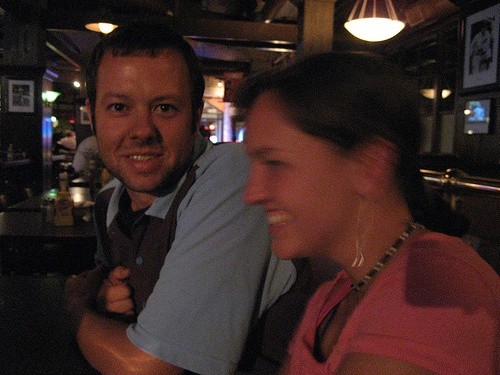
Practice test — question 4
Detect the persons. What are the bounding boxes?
[68,21,344,375]
[223,50,500,375]
[52,125,78,187]
[469,102,486,123]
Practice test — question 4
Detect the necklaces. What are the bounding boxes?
[298,221,424,336]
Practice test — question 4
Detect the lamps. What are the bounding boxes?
[344,0,405,43]
[84,14,120,35]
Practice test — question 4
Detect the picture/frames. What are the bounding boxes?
[2,75,40,119]
[455,3,500,94]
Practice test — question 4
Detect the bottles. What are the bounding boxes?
[7,143,14,161]
[53,170,74,227]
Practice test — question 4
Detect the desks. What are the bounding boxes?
[0,186,99,237]
[0,273,101,375]
